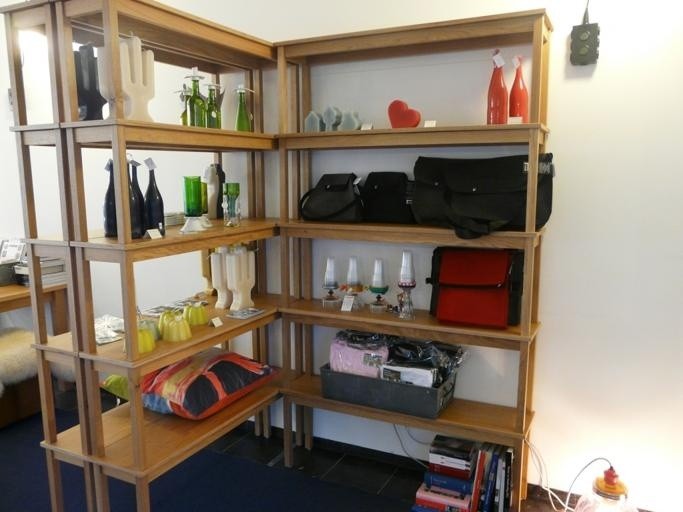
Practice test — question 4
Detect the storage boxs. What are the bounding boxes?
[320,362,457,421]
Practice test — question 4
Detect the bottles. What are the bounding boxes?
[488,51,529,125]
[179,67,254,132]
[104,159,165,242]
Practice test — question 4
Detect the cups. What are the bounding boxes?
[223,182,242,227]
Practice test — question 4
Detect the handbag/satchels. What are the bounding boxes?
[424,246,524,330]
[295,171,407,221]
[410,154,556,242]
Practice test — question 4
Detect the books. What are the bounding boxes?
[410,432,514,512]
[13,256,67,288]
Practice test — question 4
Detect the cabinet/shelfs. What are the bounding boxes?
[270,5,554,512]
[0,4,290,511]
